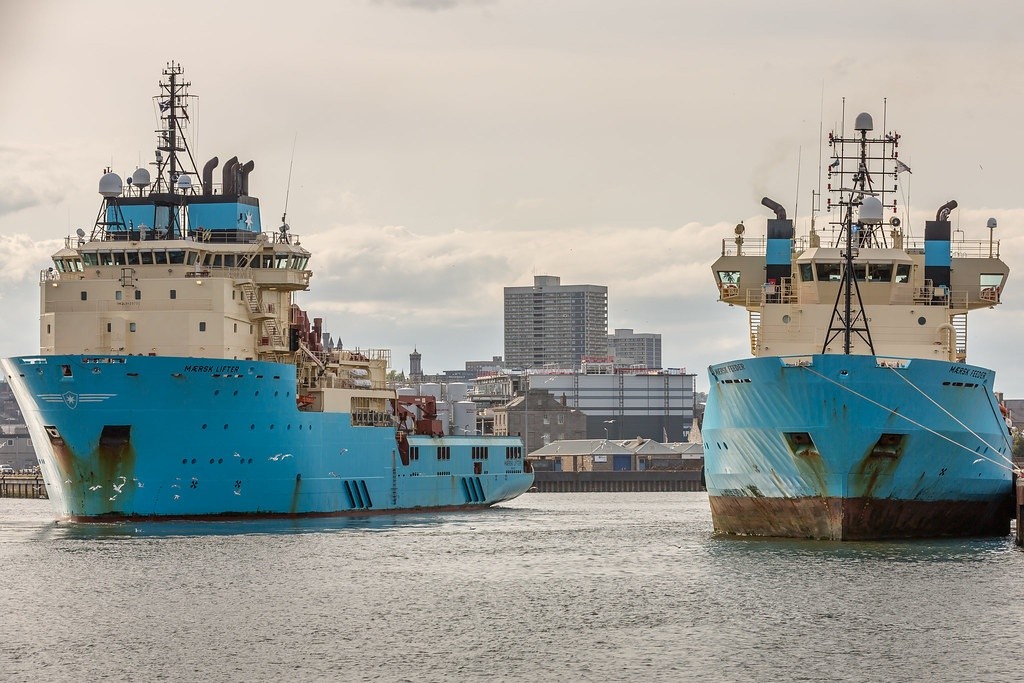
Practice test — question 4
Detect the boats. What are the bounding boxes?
[702,95,1017,544]
[0,55,536,524]
